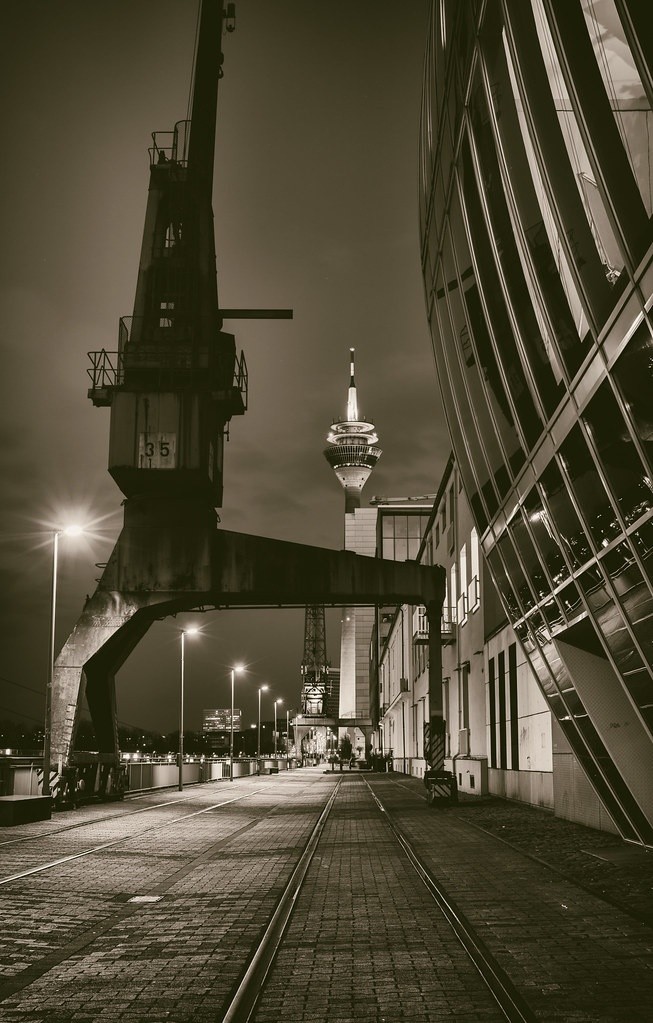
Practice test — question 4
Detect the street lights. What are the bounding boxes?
[296,714,302,751]
[286,709,293,771]
[42,528,85,795]
[274,700,282,766]
[230,666,243,781]
[258,686,268,775]
[179,628,197,791]
